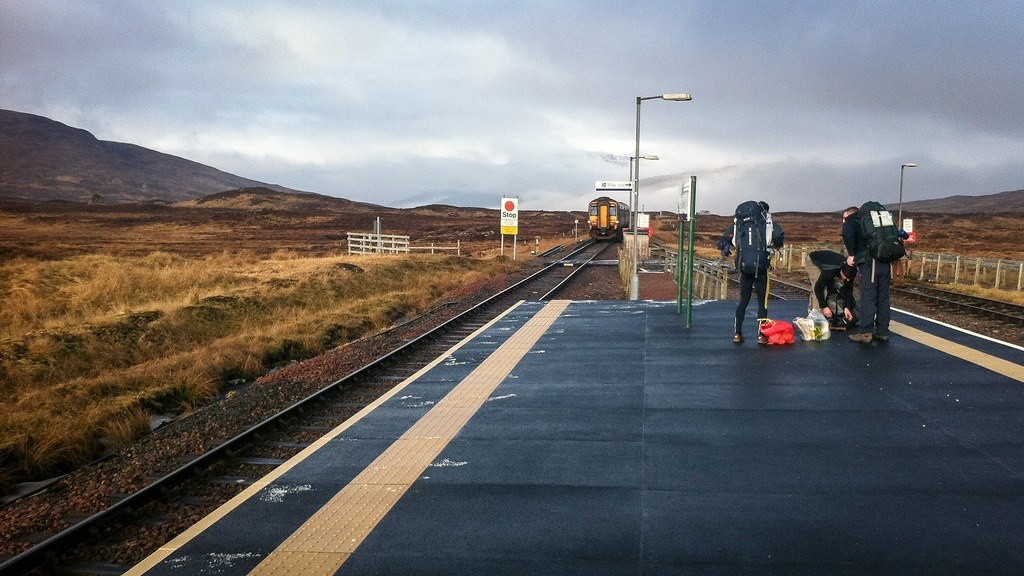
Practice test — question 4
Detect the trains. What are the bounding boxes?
[587,197,631,241]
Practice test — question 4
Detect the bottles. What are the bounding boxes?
[898,227,909,240]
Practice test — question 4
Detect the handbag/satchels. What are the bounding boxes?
[759,318,794,345]
[792,309,831,341]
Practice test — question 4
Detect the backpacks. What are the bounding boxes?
[844,201,907,262]
[732,200,773,278]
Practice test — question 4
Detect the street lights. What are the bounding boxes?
[628,155,661,233]
[628,93,693,301]
[897,163,917,231]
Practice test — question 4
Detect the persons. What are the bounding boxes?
[716,201,783,346]
[843,206,892,342]
[808,249,858,327]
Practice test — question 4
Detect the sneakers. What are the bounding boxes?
[848,332,872,344]
[732,334,745,342]
[872,333,889,341]
[757,335,768,344]
